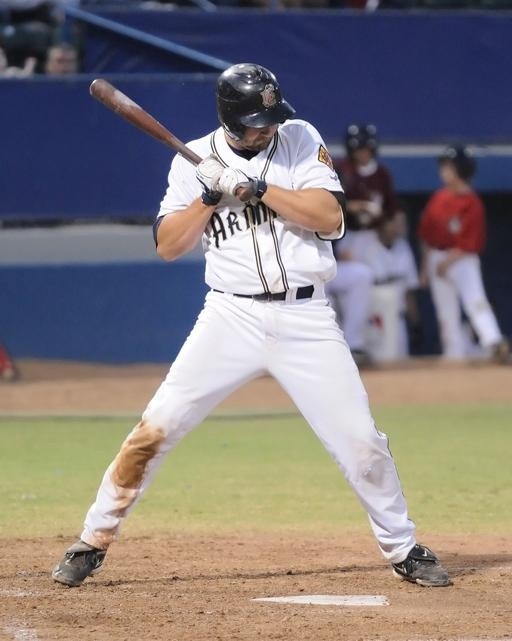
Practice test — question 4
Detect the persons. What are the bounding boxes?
[52,63,452,588]
[0,1,84,77]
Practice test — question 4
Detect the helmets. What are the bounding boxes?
[344,123,476,182]
[215,62,296,143]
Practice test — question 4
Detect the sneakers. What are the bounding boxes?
[51,538,108,588]
[391,543,451,588]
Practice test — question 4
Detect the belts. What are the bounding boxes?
[212,283,316,303]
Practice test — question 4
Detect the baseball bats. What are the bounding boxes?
[89,78,252,201]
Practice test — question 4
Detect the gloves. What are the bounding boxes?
[215,165,258,197]
[195,152,225,195]
